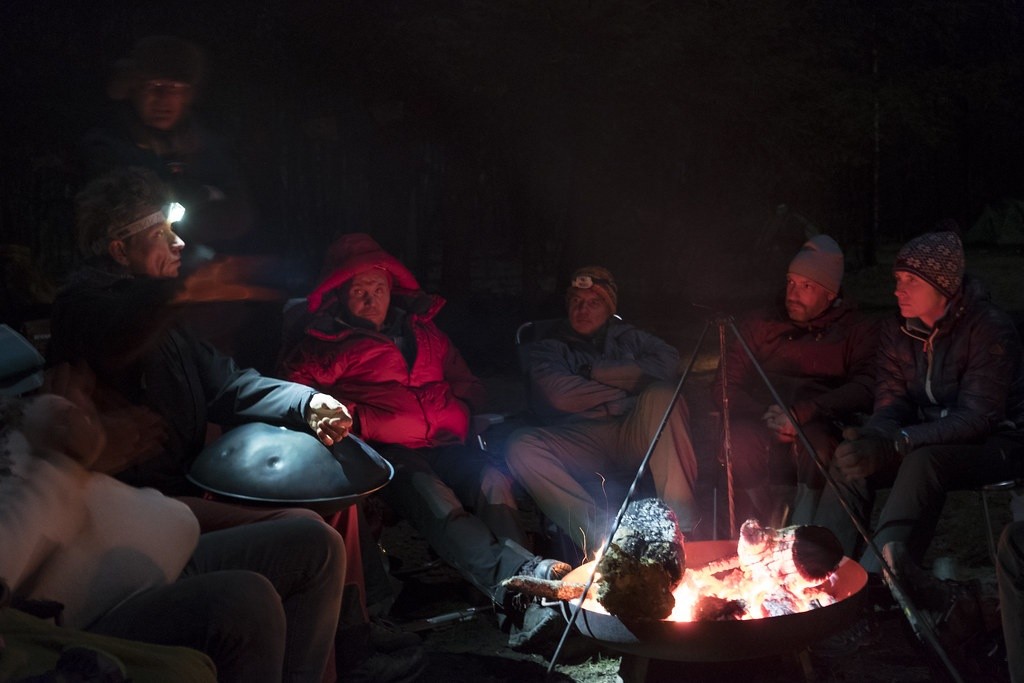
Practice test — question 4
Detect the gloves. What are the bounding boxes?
[836,426,902,483]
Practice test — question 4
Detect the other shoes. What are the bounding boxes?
[806,602,893,658]
[508,591,591,663]
[333,616,425,683]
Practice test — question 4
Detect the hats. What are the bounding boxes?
[109,35,204,102]
[892,232,964,299]
[787,235,845,297]
[567,264,617,317]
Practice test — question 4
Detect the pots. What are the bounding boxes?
[560,540,868,658]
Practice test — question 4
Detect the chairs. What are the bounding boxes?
[284,296,446,577]
[513,315,657,567]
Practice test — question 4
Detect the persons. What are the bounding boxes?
[995,520,1024,683]
[811,230,1024,658]
[712,234,881,531]
[0,37,428,683]
[281,233,572,646]
[504,264,701,566]
